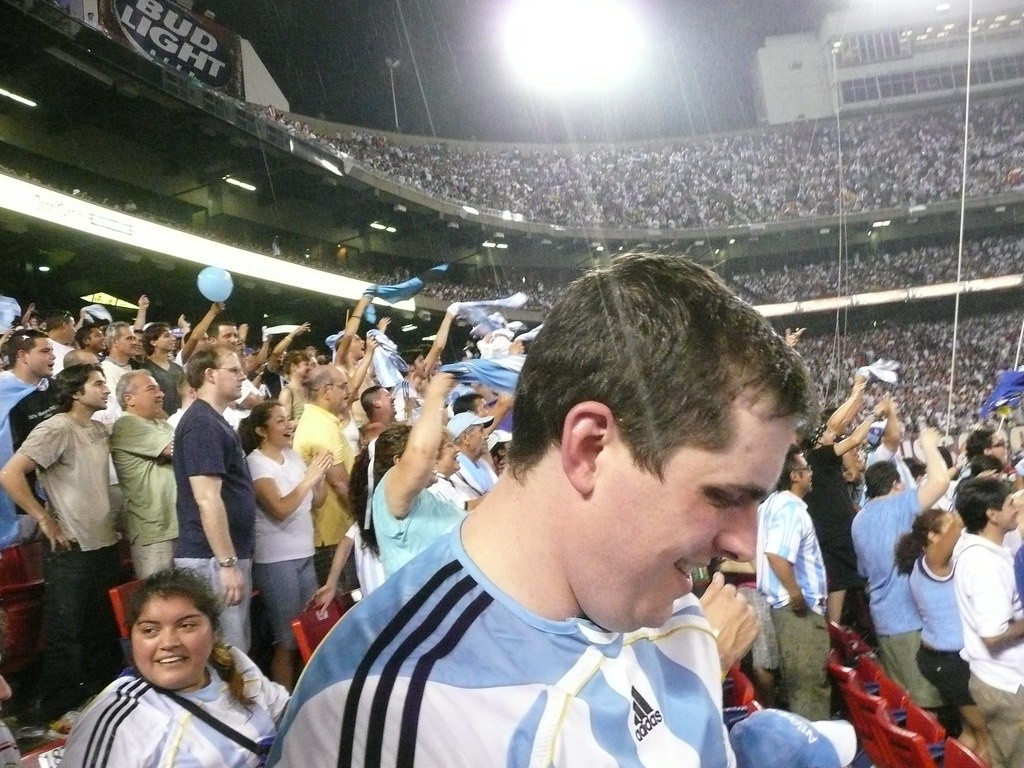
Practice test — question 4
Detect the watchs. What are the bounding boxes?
[220,556,239,567]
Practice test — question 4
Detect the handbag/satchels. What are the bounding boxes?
[256,736,275,768]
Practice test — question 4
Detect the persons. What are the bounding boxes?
[265,240,823,768]
[0,0,1023,768]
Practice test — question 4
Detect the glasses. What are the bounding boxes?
[326,383,348,390]
[22,330,38,341]
[790,465,811,473]
[64,310,72,318]
[212,367,243,377]
[991,439,1005,447]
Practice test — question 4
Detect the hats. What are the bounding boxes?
[487,430,513,451]
[447,413,494,441]
[729,708,857,768]
[867,418,887,446]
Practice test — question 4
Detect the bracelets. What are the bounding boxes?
[863,409,878,424]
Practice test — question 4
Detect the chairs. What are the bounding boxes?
[0,542,146,669]
[727,621,991,768]
[290,595,341,665]
